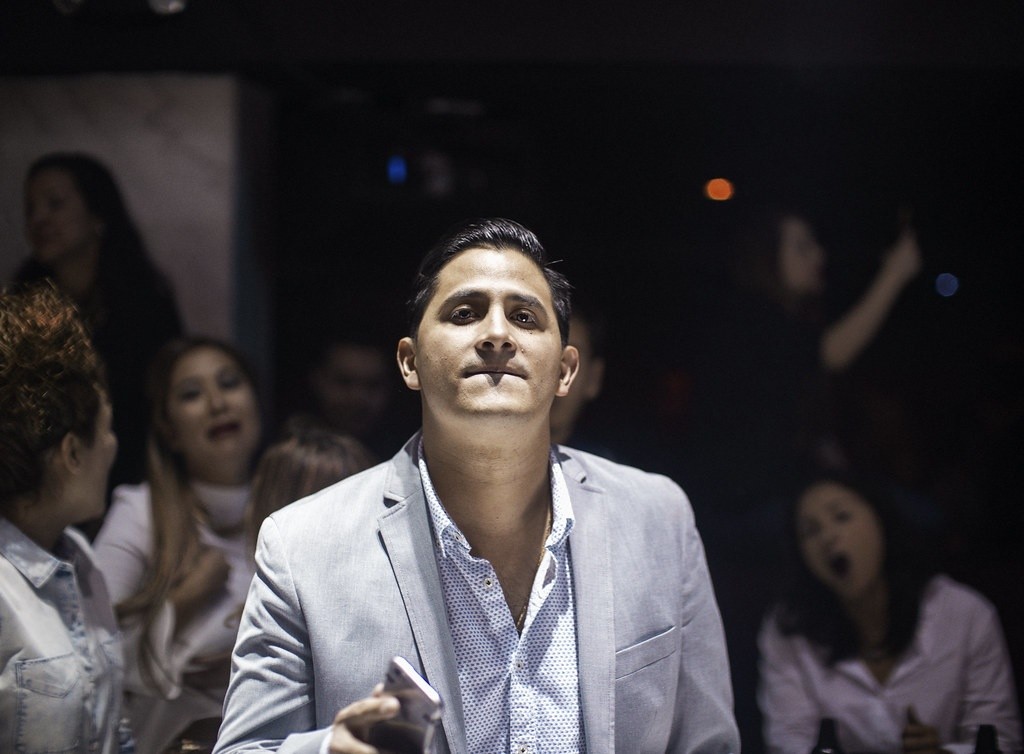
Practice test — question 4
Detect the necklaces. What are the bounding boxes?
[514,506,552,625]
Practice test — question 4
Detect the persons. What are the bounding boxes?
[214,220,742,754]
[16,151,179,505]
[0,281,601,754]
[729,205,1024,753]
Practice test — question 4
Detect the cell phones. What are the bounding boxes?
[366,657,441,754]
